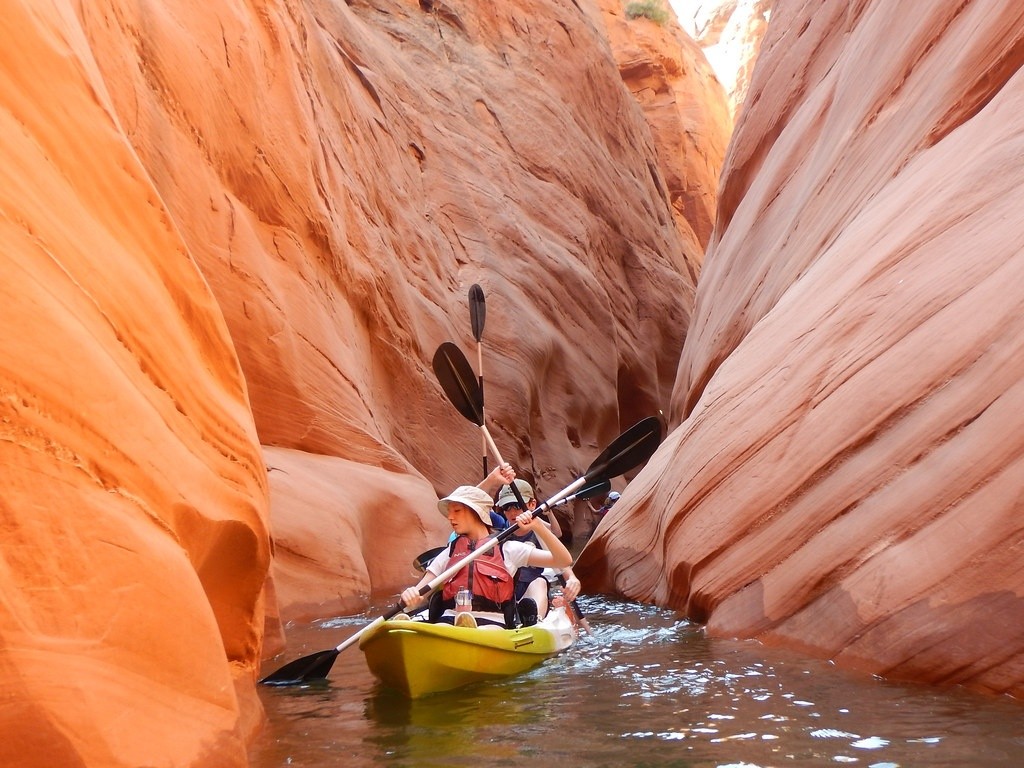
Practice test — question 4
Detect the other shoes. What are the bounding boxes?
[455,611,477,628]
[518,597,538,626]
[392,613,410,620]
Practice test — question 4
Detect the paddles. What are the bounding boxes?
[410,478,612,574]
[468,282,490,479]
[431,340,595,639]
[258,415,663,689]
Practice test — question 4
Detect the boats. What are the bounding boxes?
[357,593,579,700]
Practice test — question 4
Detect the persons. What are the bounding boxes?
[586,491,620,515]
[398,478,582,629]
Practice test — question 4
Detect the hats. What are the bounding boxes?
[499,478,534,507]
[609,491,620,501]
[495,490,503,506]
[437,486,494,526]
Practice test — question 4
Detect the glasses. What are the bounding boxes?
[502,502,530,511]
